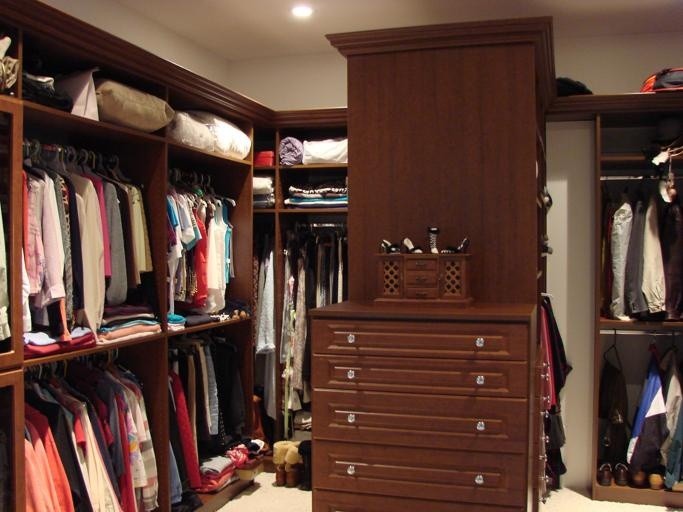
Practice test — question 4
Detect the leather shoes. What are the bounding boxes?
[599,463,664,489]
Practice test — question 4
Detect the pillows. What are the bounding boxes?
[94,78,176,133]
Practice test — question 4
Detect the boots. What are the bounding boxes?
[273,440,311,490]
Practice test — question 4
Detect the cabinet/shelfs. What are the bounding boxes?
[308,320,530,511]
[253,124,348,472]
[320,14,551,512]
[1,25,255,512]
[593,115,683,508]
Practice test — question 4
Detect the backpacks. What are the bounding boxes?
[641,68,683,91]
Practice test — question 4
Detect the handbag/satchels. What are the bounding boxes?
[61,68,101,121]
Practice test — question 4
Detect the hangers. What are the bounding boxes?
[169,165,215,193]
[22,137,121,177]
[24,347,119,385]
[610,328,675,356]
[174,331,226,349]
[603,152,677,199]
[286,222,347,248]
